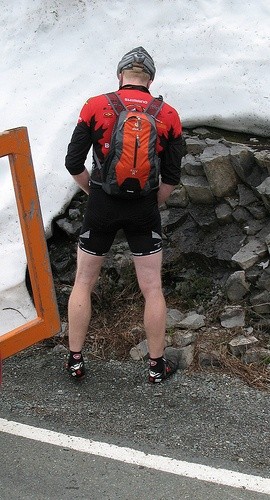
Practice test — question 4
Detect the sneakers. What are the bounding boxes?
[67,353,87,380]
[146,352,176,383]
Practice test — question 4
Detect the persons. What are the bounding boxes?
[65,46,188,383]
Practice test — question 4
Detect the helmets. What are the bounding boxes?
[116,46,156,82]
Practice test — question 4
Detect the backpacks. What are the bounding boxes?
[94,93,165,197]
